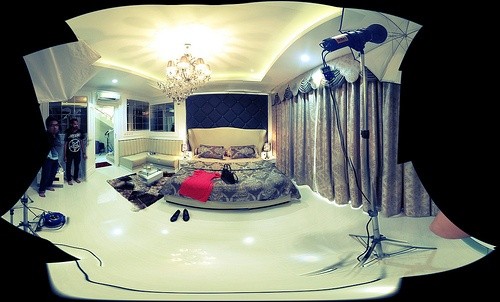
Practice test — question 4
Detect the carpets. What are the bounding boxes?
[96,162,112,169]
[106,173,175,210]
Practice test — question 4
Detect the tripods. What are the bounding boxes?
[347,43,439,278]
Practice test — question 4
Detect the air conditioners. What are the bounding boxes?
[97,90,120,102]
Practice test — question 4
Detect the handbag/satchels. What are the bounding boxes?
[221,163,238,184]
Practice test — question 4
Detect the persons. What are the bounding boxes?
[39,115,62,197]
[63,118,88,186]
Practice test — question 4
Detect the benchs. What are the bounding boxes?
[118,138,183,172]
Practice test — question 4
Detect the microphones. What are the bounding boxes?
[323,24,387,53]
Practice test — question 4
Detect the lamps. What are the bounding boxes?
[157,42,212,105]
[181,144,187,158]
[264,143,271,159]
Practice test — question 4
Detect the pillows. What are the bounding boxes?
[229,144,257,159]
[198,144,224,160]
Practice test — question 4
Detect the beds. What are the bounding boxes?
[163,127,302,210]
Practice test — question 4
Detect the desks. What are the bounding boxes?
[137,169,163,185]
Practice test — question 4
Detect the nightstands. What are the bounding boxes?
[261,155,276,165]
[178,159,189,170]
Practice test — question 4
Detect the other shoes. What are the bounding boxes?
[170,210,180,222]
[183,209,189,221]
